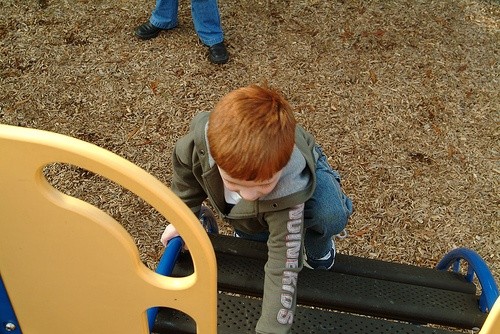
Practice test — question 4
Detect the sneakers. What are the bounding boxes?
[303,239,335,270]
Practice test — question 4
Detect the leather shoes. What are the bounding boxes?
[199,38,229,64]
[135,20,162,40]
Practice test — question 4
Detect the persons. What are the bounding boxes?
[161,84,353,334]
[135,0,229,64]
